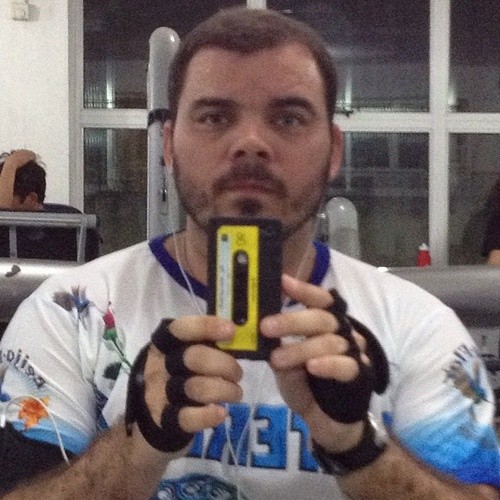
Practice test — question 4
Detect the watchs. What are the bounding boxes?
[309,411,388,477]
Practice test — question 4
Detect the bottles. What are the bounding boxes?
[417,242,430,266]
[478,336,500,424]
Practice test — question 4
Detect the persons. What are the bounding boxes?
[487,180,500,271]
[0,148,102,264]
[0,6,500,500]
